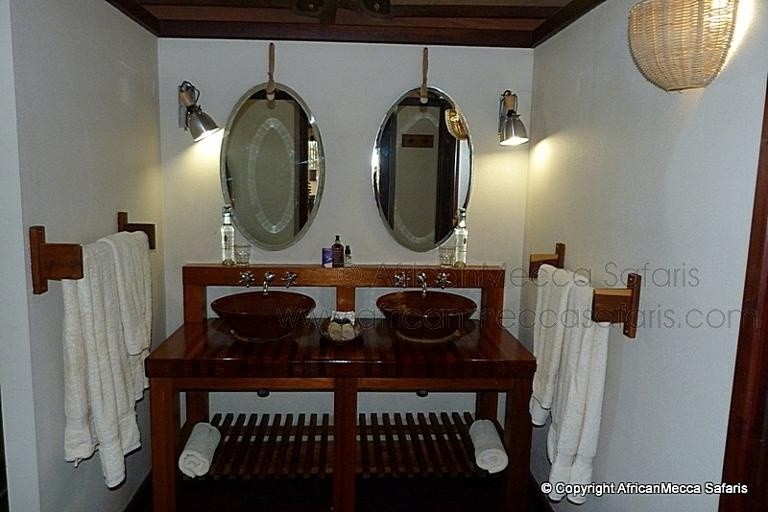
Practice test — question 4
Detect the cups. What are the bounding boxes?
[438,246,455,268]
[234,244,251,264]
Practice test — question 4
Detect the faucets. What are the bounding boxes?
[262,269,275,297]
[416,271,428,298]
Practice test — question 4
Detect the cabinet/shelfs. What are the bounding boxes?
[144,317,537,512]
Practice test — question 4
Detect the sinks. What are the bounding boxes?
[210,292,315,341]
[376,291,477,342]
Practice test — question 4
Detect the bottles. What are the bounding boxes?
[330,235,352,268]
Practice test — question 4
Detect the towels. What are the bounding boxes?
[178,422,221,478]
[60,242,143,489]
[469,420,509,474]
[97,231,153,401]
[529,264,590,426]
[547,283,611,504]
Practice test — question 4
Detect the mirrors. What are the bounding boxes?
[372,86,472,252]
[219,83,326,250]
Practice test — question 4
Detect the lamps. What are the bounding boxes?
[178,80,219,142]
[444,109,467,140]
[499,90,528,147]
